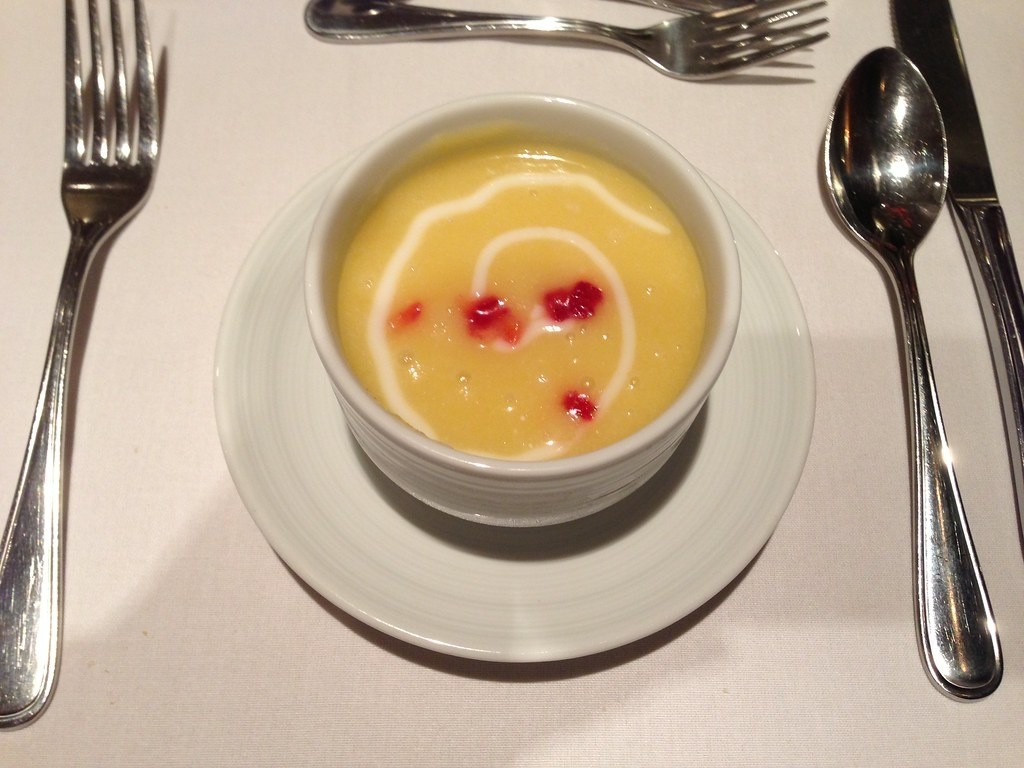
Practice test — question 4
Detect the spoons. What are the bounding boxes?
[821,46,1005,705]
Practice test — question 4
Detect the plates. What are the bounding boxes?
[211,141,817,664]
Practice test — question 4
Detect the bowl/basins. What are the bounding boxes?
[303,92,743,527]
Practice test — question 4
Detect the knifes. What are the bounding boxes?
[889,0,1024,564]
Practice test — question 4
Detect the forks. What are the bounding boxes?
[303,1,831,82]
[0,1,163,731]
